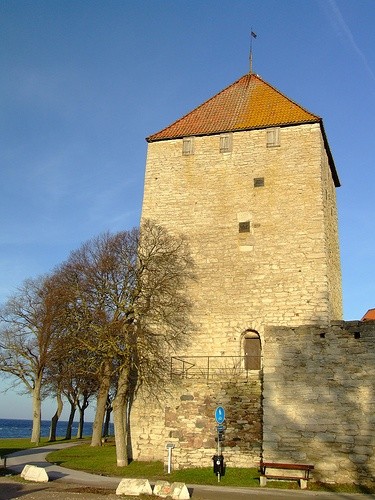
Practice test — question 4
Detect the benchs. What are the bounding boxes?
[256,461,314,490]
